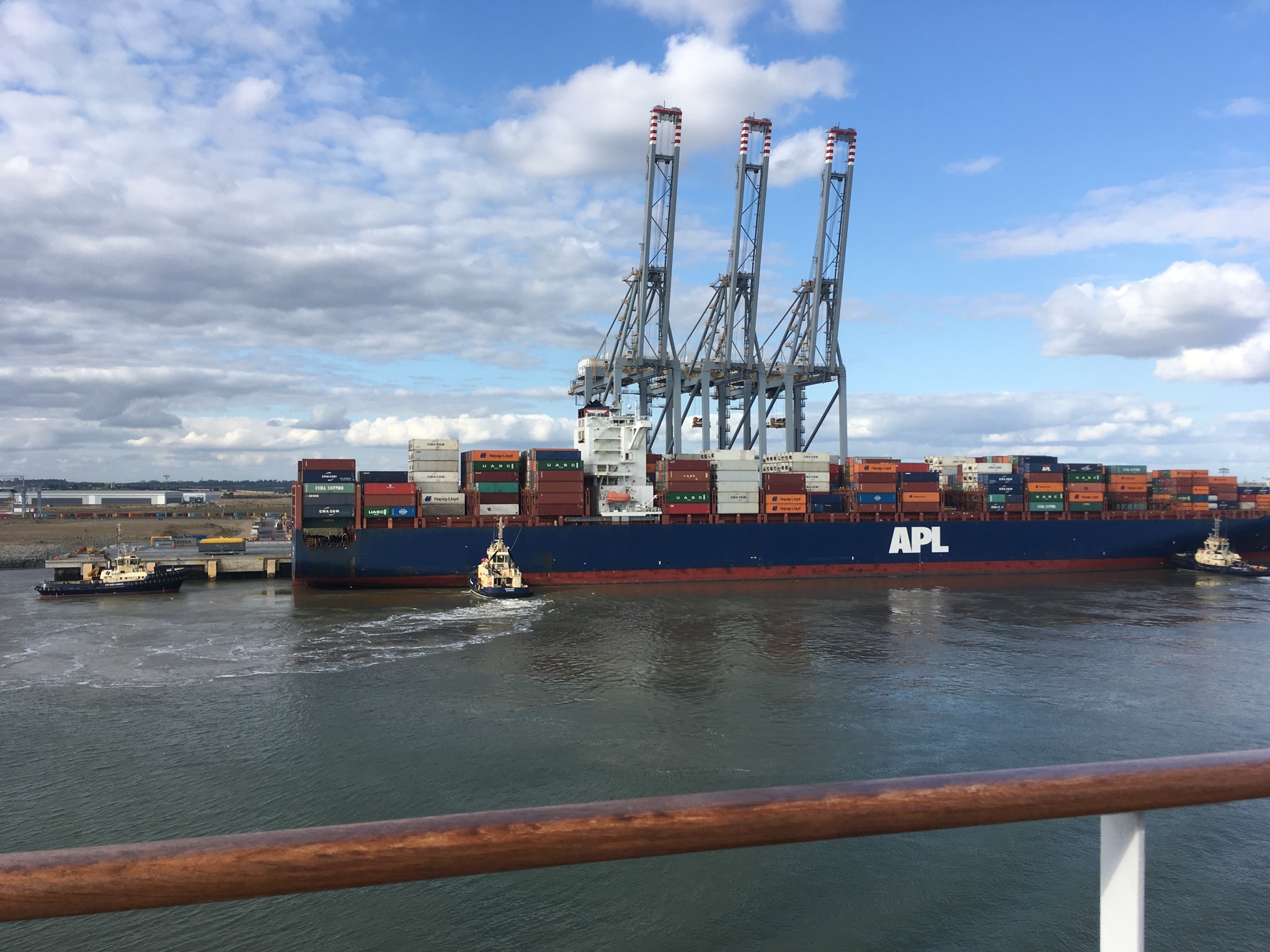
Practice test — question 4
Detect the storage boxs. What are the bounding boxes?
[292,440,1270,529]
[0,508,289,518]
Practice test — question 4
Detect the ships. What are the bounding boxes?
[293,103,1269,590]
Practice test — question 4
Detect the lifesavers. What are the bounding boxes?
[504,573,507,576]
[489,582,492,585]
[471,577,475,582]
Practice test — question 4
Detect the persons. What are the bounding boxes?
[501,581,507,587]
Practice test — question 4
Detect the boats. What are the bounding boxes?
[1175,516,1268,580]
[470,514,532,599]
[34,549,190,596]
